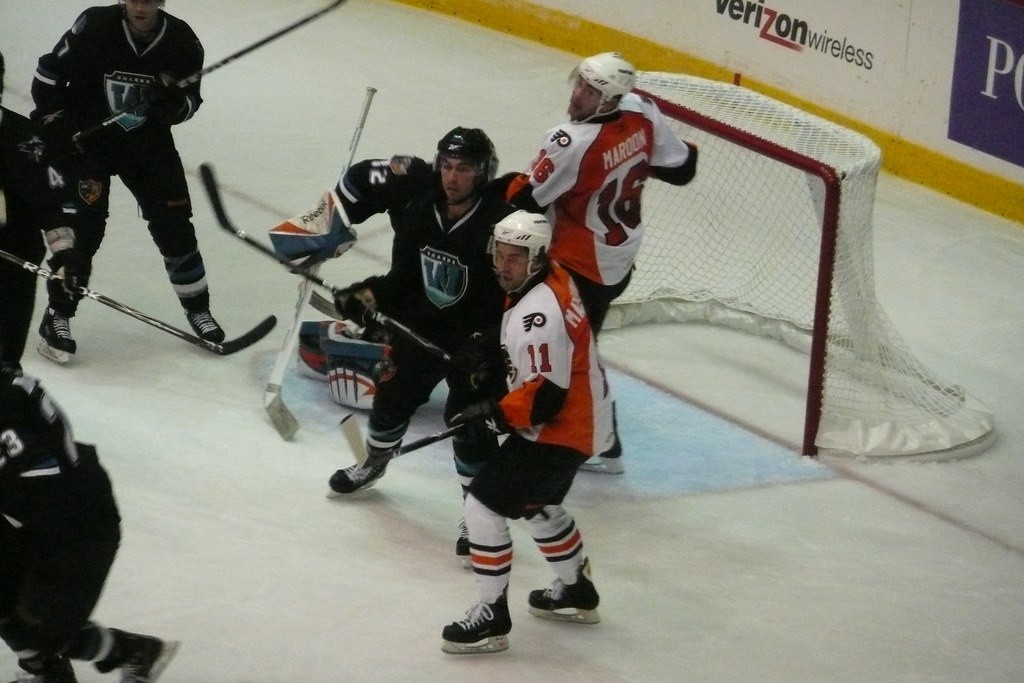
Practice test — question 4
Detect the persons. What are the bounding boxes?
[488,50,698,459]
[0,366,165,683]
[269,127,547,494]
[30,0,225,356]
[441,209,617,644]
[0,51,82,362]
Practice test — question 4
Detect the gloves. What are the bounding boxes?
[47,256,82,306]
[148,75,190,128]
[450,402,509,438]
[45,112,92,159]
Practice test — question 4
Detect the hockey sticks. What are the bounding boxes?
[2,251,278,357]
[71,0,345,139]
[360,424,470,491]
[262,85,379,441]
[198,163,452,360]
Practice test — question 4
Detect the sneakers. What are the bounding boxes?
[330,435,395,501]
[442,594,513,654]
[582,436,629,478]
[36,306,78,368]
[456,518,474,570]
[183,295,227,347]
[6,653,77,682]
[96,628,181,683]
[527,557,602,624]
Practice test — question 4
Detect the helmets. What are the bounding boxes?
[493,210,552,261]
[438,125,499,183]
[579,53,634,105]
